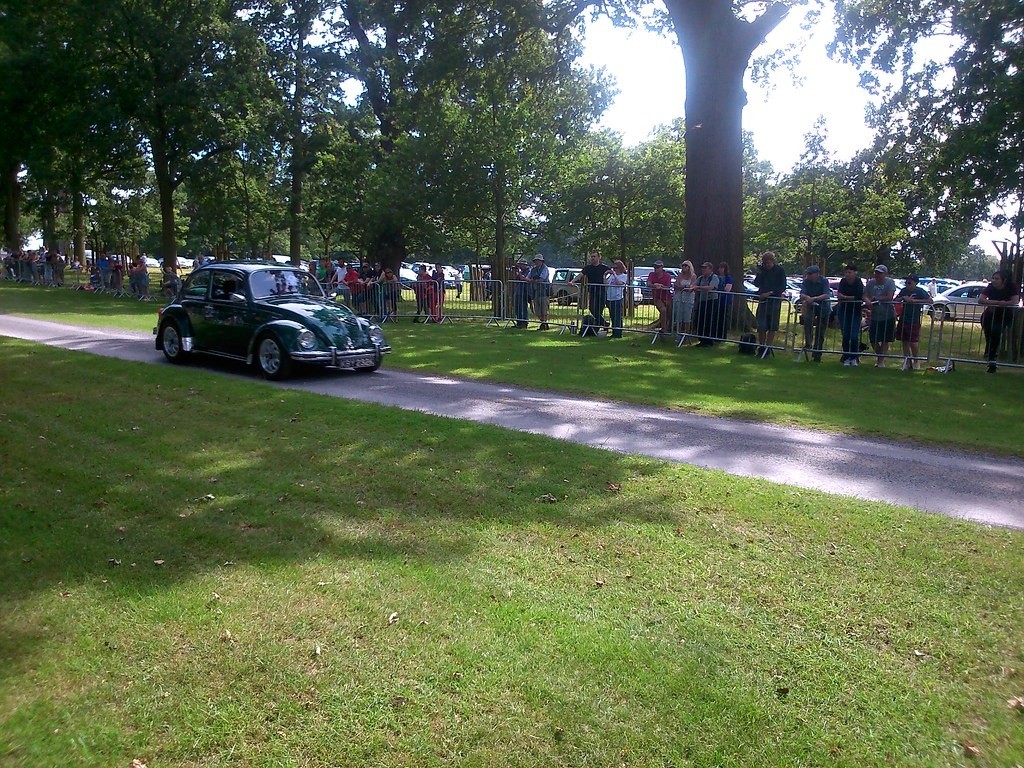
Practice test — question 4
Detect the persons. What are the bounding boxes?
[412,263,446,323]
[893,274,934,370]
[308,257,400,324]
[978,271,1019,375]
[455,268,464,298]
[159,265,174,297]
[567,251,628,338]
[928,279,937,298]
[89,252,148,299]
[192,254,209,273]
[647,260,672,333]
[511,254,554,331]
[753,251,787,359]
[800,266,831,363]
[863,265,896,368]
[0,246,80,285]
[482,268,492,300]
[837,265,865,367]
[673,260,733,347]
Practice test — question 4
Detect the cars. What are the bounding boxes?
[152,257,392,379]
[920,281,1024,323]
[630,265,959,312]
[79,248,546,288]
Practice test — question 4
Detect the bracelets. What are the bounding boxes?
[768,291,774,295]
[685,285,686,288]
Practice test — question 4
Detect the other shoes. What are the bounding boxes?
[904,359,911,369]
[912,362,921,370]
[985,363,997,373]
[511,321,885,368]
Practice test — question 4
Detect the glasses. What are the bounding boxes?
[346,268,351,269]
[654,265,662,268]
[682,264,690,268]
[807,273,813,275]
[905,282,915,285]
[386,271,392,274]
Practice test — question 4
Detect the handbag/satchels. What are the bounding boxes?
[579,315,596,336]
[738,334,757,355]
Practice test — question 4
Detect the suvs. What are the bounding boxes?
[549,267,644,307]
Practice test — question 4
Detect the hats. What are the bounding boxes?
[653,260,664,266]
[362,263,370,268]
[805,265,820,273]
[700,262,713,270]
[517,259,529,265]
[874,265,888,273]
[533,254,544,264]
[903,273,920,283]
[844,263,855,270]
[375,262,380,266]
